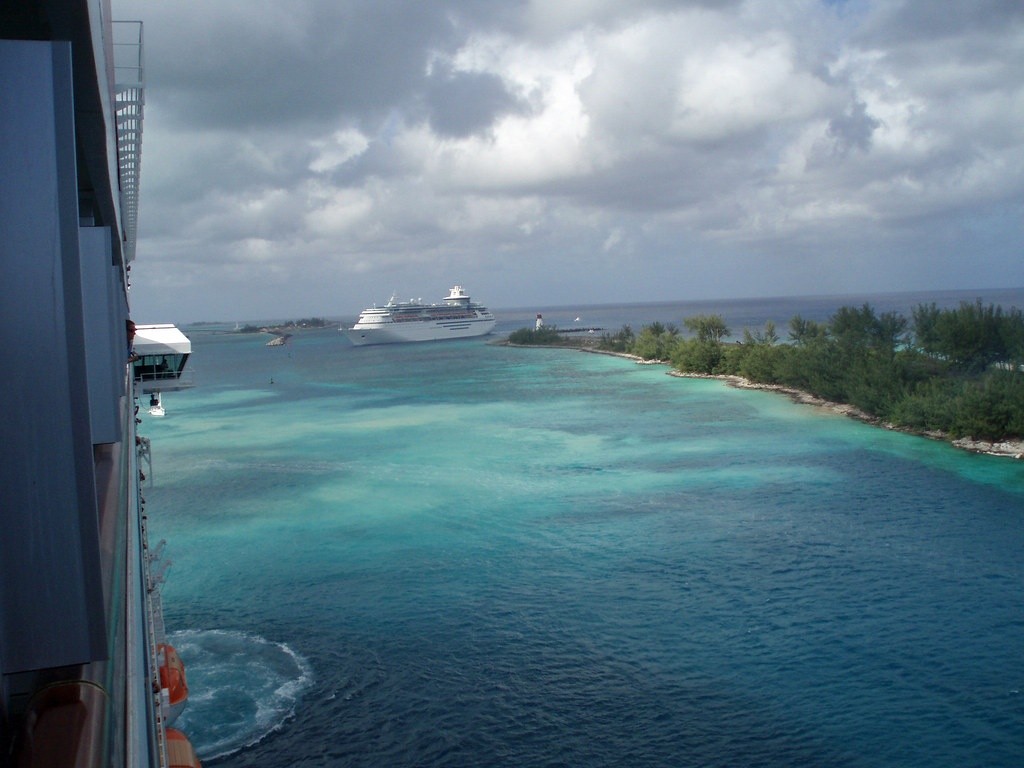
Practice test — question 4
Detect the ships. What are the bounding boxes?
[336,284,498,346]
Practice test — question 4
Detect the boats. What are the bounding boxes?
[148,393,165,417]
[132,322,198,394]
